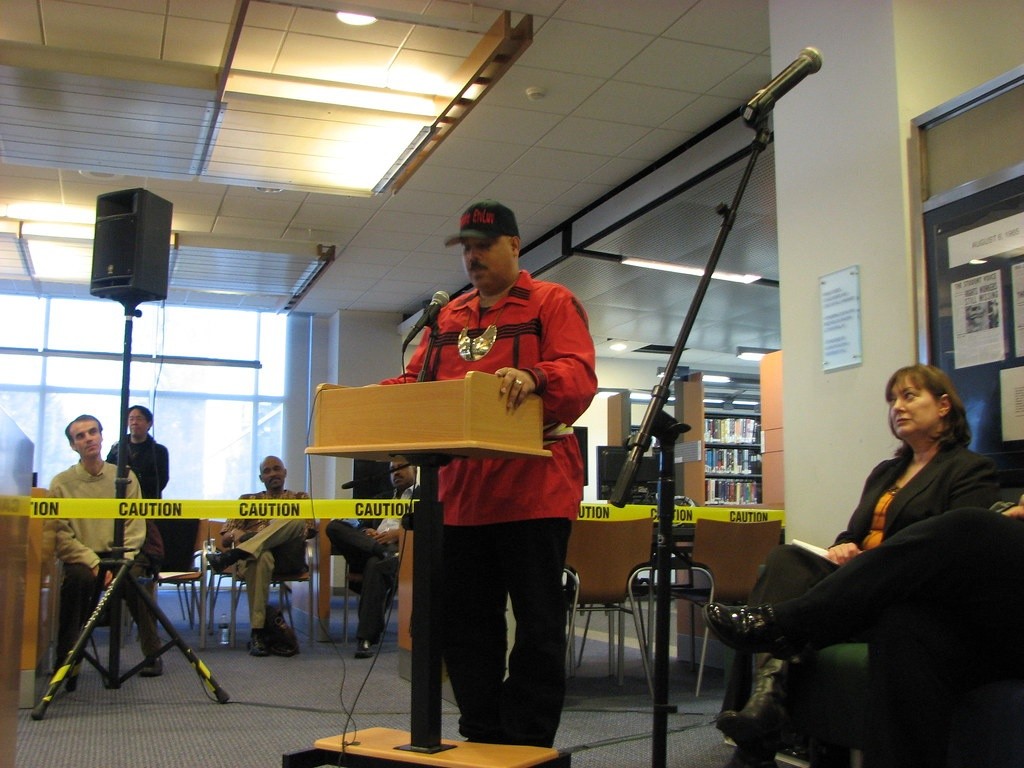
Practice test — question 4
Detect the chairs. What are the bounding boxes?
[562,516,782,703]
[119,517,400,649]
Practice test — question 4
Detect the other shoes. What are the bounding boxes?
[355,641,375,658]
[207,553,228,572]
[63,677,77,692]
[374,544,390,561]
[251,635,267,656]
[142,657,163,676]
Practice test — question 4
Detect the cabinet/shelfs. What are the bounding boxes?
[674,372,763,507]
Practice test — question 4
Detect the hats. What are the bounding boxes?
[445,198,520,246]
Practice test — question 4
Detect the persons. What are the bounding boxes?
[702,490,1024,768]
[358,198,599,748]
[325,460,420,658]
[50,415,163,676]
[700,366,1000,768]
[106,404,168,501]
[206,456,316,656]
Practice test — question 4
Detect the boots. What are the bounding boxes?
[718,656,798,756]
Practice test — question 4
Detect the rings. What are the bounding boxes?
[515,380,522,385]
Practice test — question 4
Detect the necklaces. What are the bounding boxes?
[886,485,901,495]
[457,306,502,361]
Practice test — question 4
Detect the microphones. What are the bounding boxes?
[403,290,450,351]
[742,46,823,123]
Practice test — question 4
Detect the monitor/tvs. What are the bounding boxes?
[597,445,660,506]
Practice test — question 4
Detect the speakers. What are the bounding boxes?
[90,187,174,303]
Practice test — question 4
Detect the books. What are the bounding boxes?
[703,418,762,504]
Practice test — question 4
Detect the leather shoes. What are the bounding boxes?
[703,602,781,656]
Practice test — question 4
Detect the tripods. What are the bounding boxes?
[31,302,230,722]
